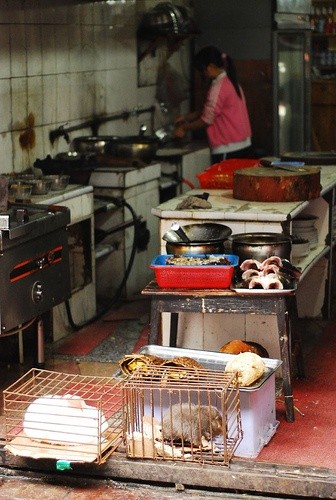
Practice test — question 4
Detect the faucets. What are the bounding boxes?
[160,102,168,113]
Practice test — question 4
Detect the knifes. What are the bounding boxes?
[259,159,297,172]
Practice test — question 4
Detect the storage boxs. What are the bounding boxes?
[128,370,281,460]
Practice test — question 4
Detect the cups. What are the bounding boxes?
[10,184,33,203]
[0,176,9,212]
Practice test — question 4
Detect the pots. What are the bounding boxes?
[55,135,159,171]
[162,221,293,268]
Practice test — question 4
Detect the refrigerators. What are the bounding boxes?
[192,0,314,156]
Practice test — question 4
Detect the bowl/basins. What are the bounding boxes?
[21,179,55,195]
[292,240,310,259]
[41,175,71,191]
[153,122,178,146]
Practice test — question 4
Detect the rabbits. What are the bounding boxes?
[161,403,227,449]
[22,393,109,446]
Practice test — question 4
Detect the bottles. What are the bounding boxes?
[313,43,336,66]
[310,5,334,33]
[293,213,320,248]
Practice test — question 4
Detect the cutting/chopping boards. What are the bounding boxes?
[234,165,321,202]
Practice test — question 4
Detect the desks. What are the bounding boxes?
[140,278,297,422]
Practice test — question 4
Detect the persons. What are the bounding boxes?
[171,44,252,165]
